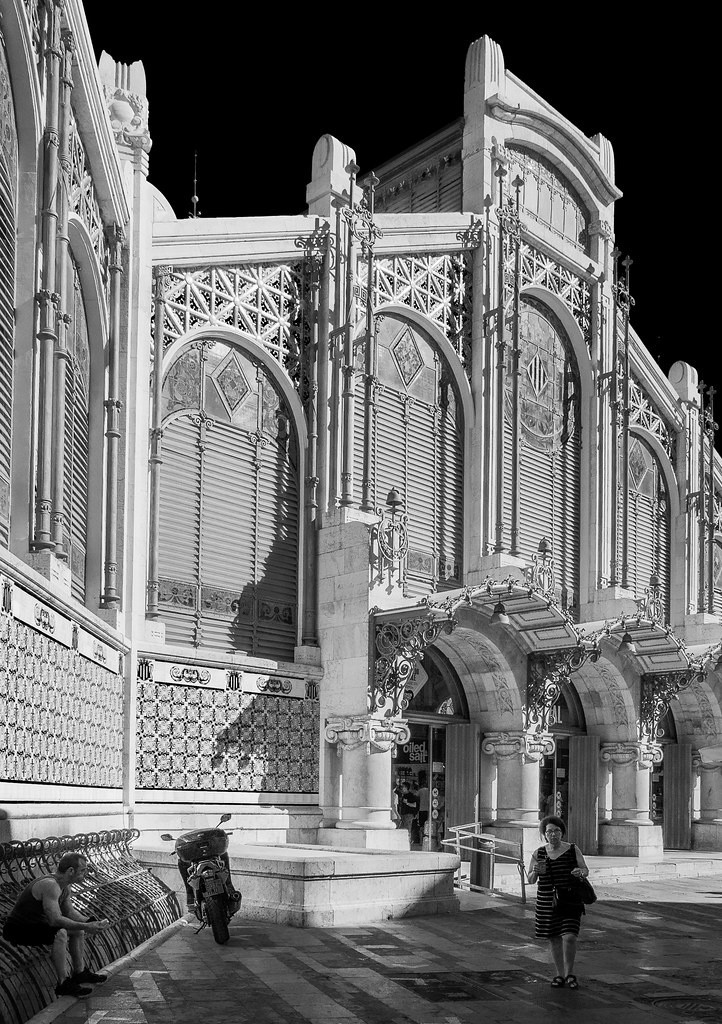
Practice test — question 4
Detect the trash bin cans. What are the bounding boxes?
[471,833,497,890]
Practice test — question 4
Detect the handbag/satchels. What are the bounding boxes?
[571,842,597,904]
[551,884,587,918]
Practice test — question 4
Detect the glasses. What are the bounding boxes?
[545,828,562,834]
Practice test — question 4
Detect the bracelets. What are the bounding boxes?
[581,870,584,876]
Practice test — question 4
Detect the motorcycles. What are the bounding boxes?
[159,813,242,946]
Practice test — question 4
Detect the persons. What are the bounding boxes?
[3,852,108,996]
[527,816,589,989]
[391,779,429,846]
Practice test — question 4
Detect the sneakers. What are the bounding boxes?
[55,977,92,997]
[70,967,107,984]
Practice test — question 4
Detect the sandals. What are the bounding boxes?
[566,974,578,990]
[551,975,565,987]
